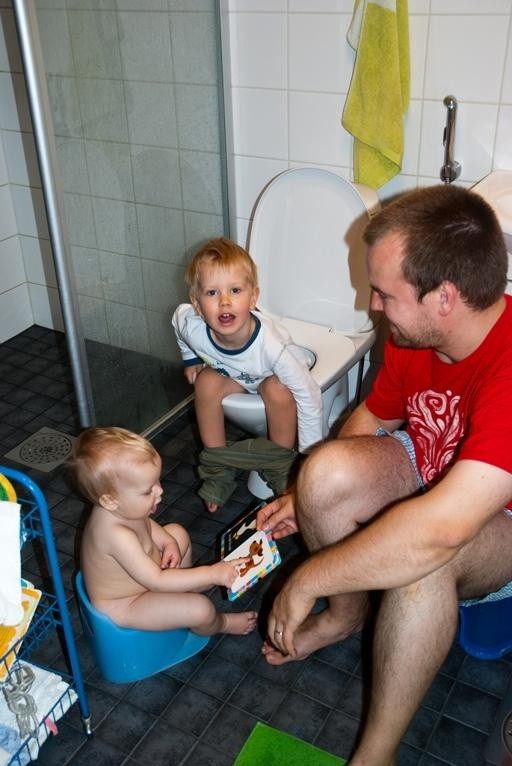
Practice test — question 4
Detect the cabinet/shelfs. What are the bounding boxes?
[0,467,98,766]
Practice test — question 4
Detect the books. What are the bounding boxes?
[220,500,282,602]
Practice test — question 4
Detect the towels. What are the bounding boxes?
[339,2,412,193]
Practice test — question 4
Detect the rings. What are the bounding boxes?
[275,630,283,636]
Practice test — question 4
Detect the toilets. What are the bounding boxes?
[222,167,383,442]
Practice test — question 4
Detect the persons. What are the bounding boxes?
[169,237,325,511]
[75,426,259,636]
[257,185,512,765]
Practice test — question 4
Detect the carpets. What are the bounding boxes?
[230,717,348,766]
